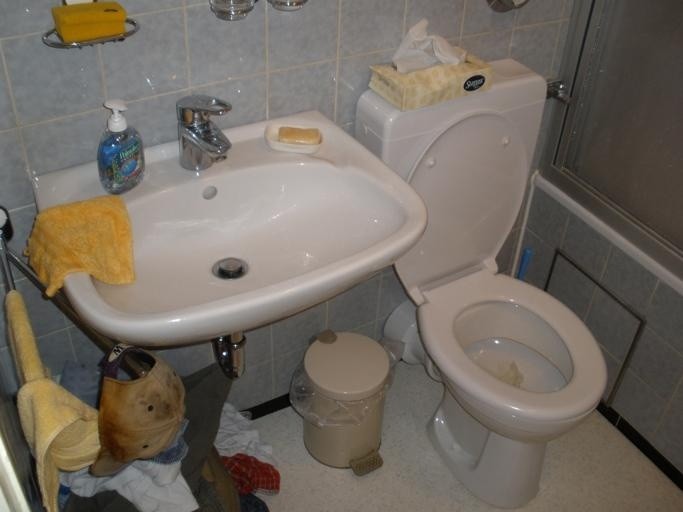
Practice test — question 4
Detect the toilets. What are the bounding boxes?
[349,54,610,512]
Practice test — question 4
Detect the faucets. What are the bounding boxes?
[174,92,233,173]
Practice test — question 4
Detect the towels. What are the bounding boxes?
[22,194,134,297]
[4,289,103,511]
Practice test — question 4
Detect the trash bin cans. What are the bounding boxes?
[302,328,393,477]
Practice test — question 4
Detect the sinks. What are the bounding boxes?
[24,106,433,348]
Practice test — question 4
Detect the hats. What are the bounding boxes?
[88,341,186,478]
